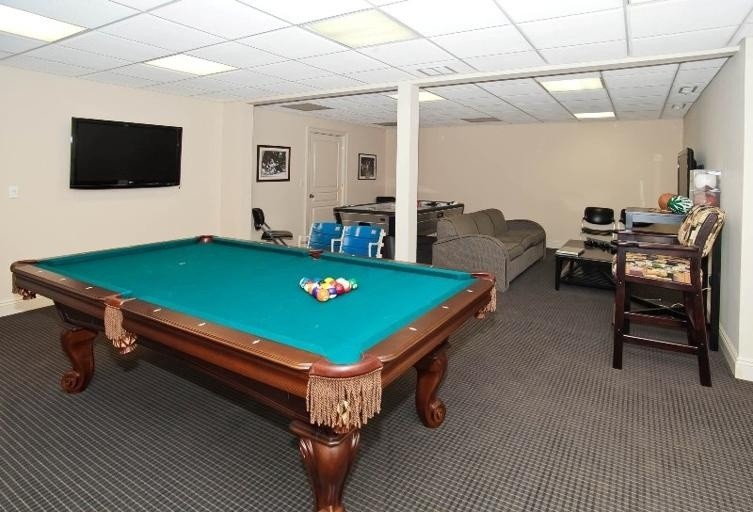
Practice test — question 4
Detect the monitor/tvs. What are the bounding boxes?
[70,116,183,189]
[677,147,697,198]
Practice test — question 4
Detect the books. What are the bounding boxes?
[554,246,584,257]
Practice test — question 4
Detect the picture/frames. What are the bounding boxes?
[357,153,377,180]
[256,145,290,183]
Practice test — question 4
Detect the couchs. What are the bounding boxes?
[430,208,546,293]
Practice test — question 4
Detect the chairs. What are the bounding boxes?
[611,204,725,387]
[297,221,386,259]
[579,207,654,238]
[251,208,293,245]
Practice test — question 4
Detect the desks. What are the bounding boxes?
[9,235,496,510]
[333,200,464,235]
[624,208,685,234]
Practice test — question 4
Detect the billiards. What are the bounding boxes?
[300,277,357,301]
